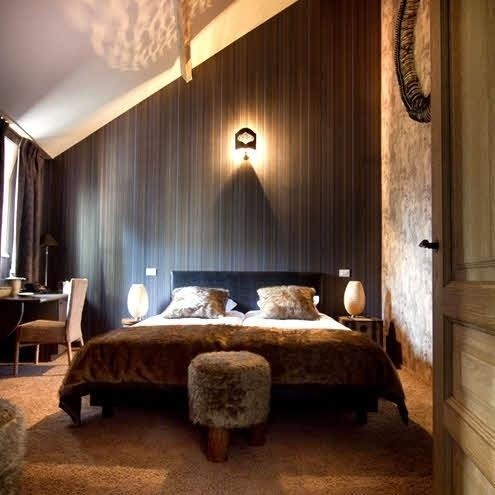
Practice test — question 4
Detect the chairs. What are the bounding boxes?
[13,278,90,377]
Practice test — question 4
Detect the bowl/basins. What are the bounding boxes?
[0,287,12,298]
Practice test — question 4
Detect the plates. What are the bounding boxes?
[18,292,34,297]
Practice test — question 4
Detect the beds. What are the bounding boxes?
[92,270,381,425]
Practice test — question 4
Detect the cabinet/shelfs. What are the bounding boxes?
[0,293,68,363]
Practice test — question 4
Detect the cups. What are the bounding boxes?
[5,280,20,296]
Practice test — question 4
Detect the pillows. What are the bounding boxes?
[160,284,322,321]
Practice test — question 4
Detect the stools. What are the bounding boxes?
[186,349,273,463]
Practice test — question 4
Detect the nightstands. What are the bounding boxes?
[338,315,384,347]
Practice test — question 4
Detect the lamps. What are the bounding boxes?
[343,280,367,318]
[126,283,149,322]
[234,126,258,161]
[39,232,58,293]
